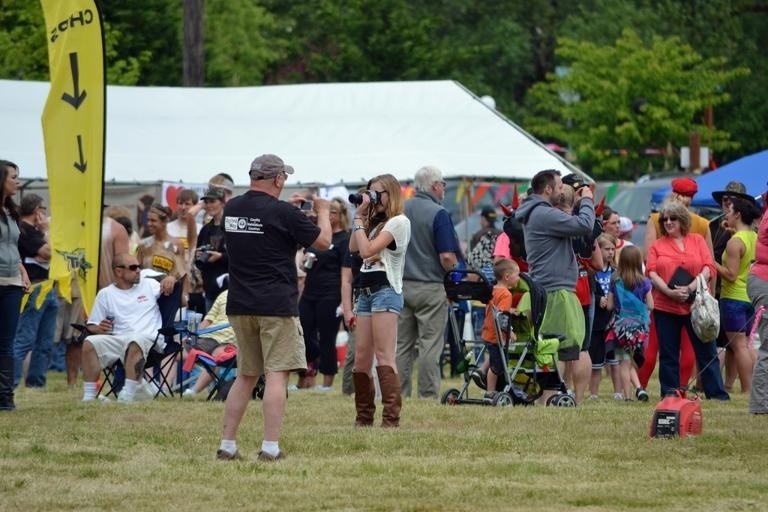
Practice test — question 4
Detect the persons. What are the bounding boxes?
[215,156,332,460]
[0,155,767,426]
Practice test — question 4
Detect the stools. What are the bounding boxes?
[194,350,241,401]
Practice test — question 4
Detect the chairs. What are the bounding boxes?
[96,272,187,400]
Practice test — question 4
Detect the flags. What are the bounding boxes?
[40,1,106,317]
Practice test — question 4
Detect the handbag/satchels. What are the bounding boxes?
[689,273,721,343]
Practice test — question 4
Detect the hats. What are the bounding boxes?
[712,181,755,205]
[201,188,227,202]
[248,154,294,180]
[671,177,698,197]
[480,206,497,220]
[561,174,590,195]
[619,217,639,236]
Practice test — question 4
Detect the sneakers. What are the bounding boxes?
[472,370,487,390]
[483,391,498,401]
[215,449,243,461]
[288,385,334,392]
[258,450,285,462]
[558,387,648,401]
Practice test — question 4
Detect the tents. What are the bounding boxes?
[0,77,595,231]
[651,150,767,219]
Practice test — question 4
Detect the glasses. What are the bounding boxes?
[661,215,678,221]
[113,265,141,270]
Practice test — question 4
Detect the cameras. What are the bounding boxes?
[193,249,219,270]
[297,201,315,212]
[349,190,381,207]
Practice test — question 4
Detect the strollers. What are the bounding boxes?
[437,270,575,407]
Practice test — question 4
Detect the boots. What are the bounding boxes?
[351,370,375,427]
[375,366,402,428]
